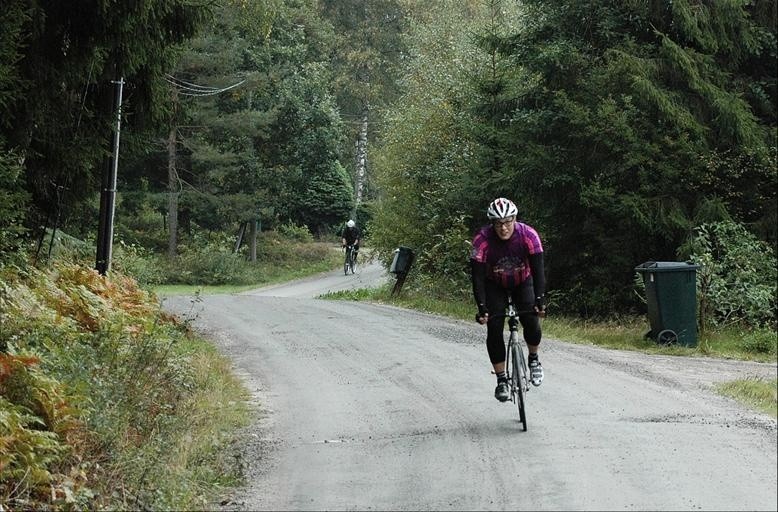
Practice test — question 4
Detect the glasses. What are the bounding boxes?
[494,218,514,226]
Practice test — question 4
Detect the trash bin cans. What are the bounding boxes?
[634,260,700,348]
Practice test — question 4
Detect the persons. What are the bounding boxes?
[469,197,546,402]
[342,219,360,261]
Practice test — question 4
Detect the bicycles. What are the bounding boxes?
[479,296,543,431]
[341,244,357,275]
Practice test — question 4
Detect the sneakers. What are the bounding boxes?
[495,378,509,402]
[528,360,544,386]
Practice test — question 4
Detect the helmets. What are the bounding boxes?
[347,219,355,228]
[487,198,519,219]
